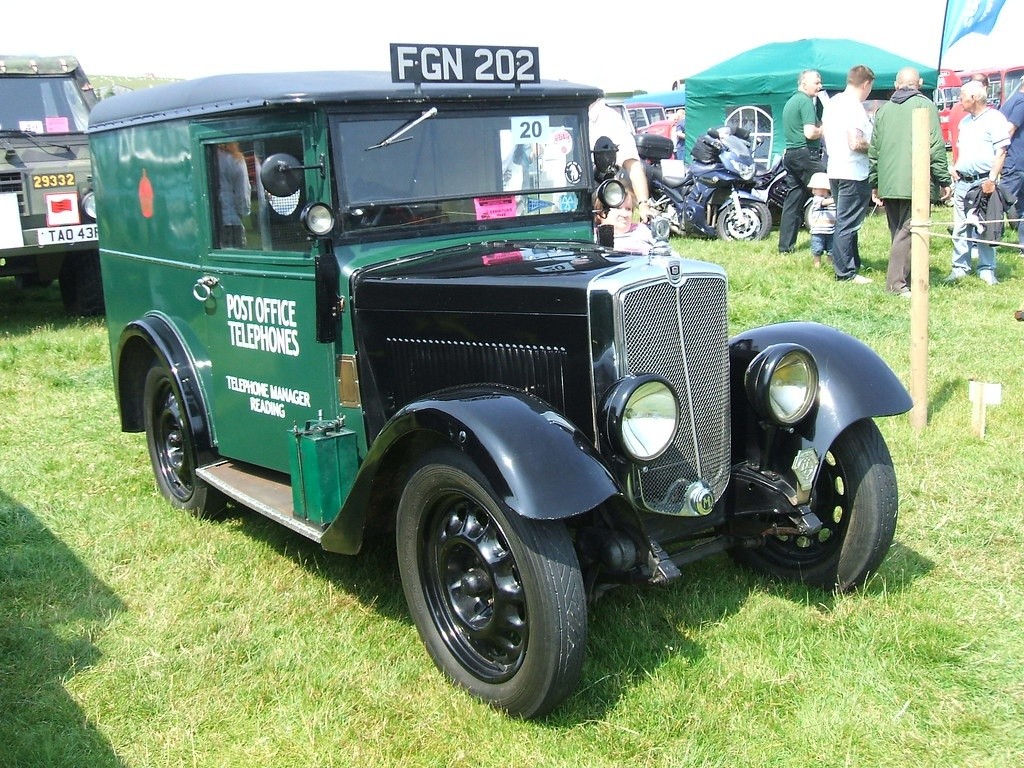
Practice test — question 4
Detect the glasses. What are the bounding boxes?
[957,97,968,102]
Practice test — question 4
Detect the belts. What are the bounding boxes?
[956,171,990,182]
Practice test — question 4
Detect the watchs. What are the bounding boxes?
[988,175,996,183]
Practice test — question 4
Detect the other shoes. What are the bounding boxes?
[851,275,873,285]
[952,267,965,278]
[901,291,911,297]
[980,269,999,286]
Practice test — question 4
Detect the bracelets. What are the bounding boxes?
[639,200,647,204]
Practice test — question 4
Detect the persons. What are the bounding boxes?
[824,65,874,285]
[216,142,253,249]
[948,73,988,167]
[940,82,1011,285]
[806,173,836,269]
[584,89,653,254]
[997,82,1024,320]
[869,66,952,297]
[672,109,685,161]
[779,70,823,254]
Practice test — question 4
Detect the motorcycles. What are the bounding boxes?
[633,128,772,242]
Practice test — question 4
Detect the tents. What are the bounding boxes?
[684,36,940,171]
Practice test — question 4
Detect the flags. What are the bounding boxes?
[942,0,1005,52]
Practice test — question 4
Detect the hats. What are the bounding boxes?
[807,172,831,189]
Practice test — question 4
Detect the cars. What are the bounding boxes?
[82,43,915,723]
[606,91,685,159]
[0,56,105,316]
[932,67,1023,148]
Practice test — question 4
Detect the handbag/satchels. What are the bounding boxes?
[690,136,721,164]
